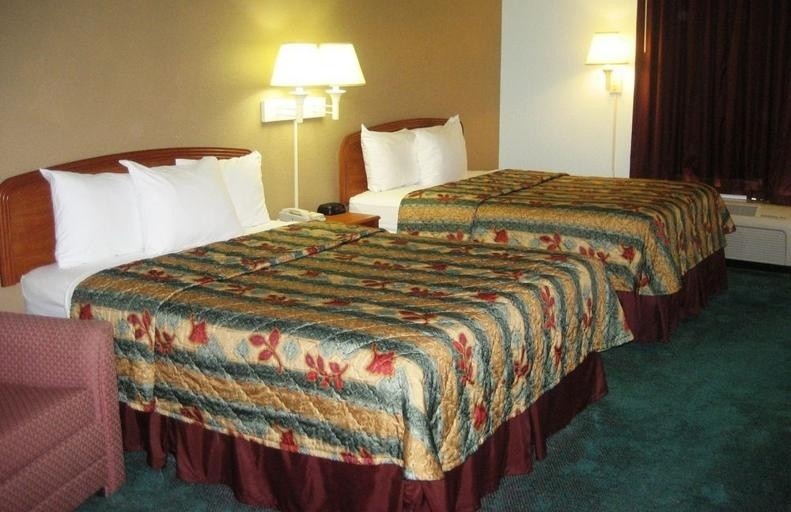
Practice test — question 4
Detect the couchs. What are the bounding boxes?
[0,311,125,512]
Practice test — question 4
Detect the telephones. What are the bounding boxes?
[279,207,326,223]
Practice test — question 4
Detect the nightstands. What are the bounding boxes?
[324,212,381,228]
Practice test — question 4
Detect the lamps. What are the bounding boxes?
[583,31,633,93]
[320,43,366,120]
[269,42,329,124]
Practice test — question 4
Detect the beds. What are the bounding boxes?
[0,147,634,512]
[338,118,736,340]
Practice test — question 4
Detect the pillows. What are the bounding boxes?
[175,151,272,234]
[360,123,419,192]
[118,155,244,258]
[413,113,468,188]
[38,168,143,269]
